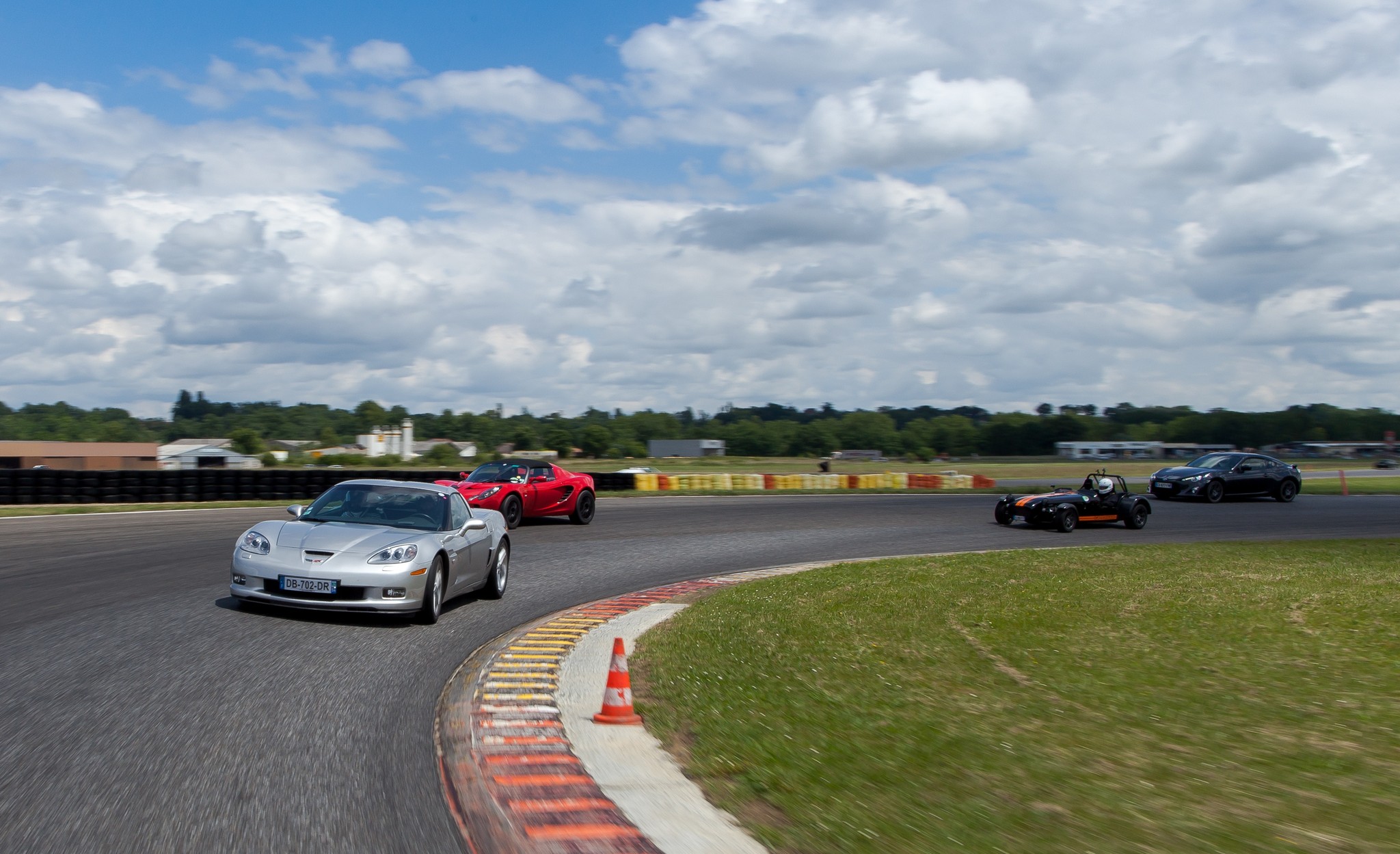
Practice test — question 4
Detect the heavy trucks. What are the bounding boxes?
[831,449,882,460]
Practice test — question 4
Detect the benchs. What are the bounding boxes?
[377,500,418,521]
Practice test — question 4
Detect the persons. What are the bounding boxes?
[1097,478,1118,505]
[408,494,444,525]
[338,489,382,520]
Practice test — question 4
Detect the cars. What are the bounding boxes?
[301,463,318,468]
[327,464,346,468]
[1374,459,1400,469]
[1147,451,1303,503]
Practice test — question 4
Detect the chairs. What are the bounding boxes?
[1077,479,1095,492]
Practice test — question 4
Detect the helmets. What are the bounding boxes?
[1098,478,1113,494]
[497,465,515,478]
[345,489,371,517]
[416,494,438,518]
[1218,455,1230,468]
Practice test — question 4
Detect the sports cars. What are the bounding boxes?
[430,458,596,529]
[994,467,1151,533]
[229,478,511,624]
